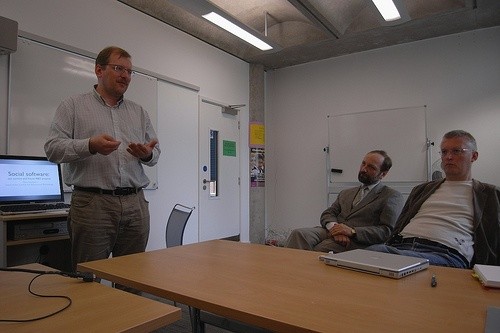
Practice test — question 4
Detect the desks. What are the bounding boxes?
[0,262,183,333]
[0,211,72,268]
[75,239,500,333]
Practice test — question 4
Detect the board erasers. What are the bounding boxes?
[331,169,342,173]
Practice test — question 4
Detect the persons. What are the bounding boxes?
[284,150,404,255]
[44,46,161,296]
[365,130,500,270]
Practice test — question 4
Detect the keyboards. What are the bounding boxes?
[0,203,71,214]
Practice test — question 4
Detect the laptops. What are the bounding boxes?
[319,249,430,278]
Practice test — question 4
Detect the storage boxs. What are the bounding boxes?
[10,221,69,241]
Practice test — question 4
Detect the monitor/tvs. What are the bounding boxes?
[0,155,64,204]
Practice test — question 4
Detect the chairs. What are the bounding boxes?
[165,204,196,306]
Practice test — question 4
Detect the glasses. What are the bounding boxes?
[439,148,469,155]
[101,63,135,77]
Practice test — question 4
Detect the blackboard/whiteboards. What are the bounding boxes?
[327,105,429,183]
[7,37,159,191]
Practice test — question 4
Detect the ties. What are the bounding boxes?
[352,186,366,208]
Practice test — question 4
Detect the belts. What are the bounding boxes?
[73,185,143,195]
[394,237,470,267]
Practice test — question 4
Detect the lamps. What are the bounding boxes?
[167,0,285,56]
[370,0,412,28]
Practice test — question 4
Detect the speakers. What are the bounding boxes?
[0,16,18,55]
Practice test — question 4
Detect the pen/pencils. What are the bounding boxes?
[431,274,437,287]
